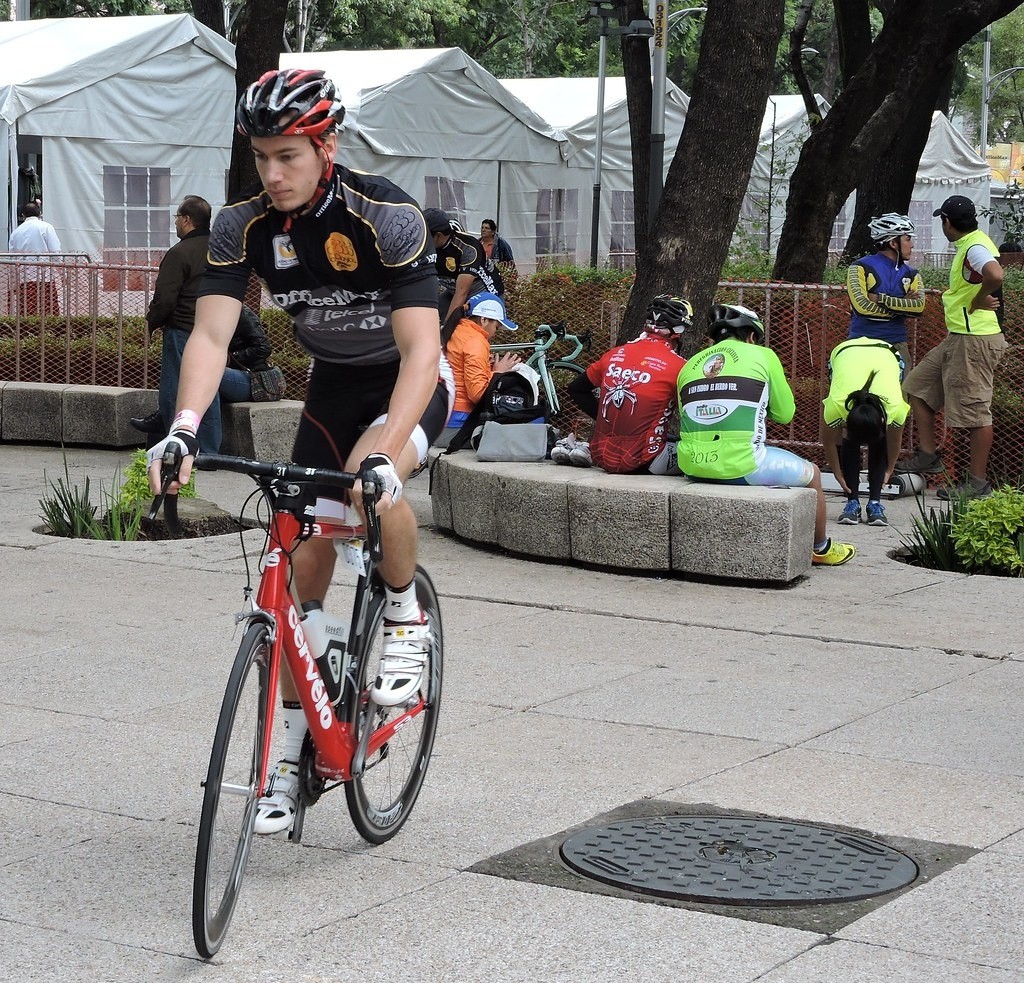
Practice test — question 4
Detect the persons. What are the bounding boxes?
[901,195,1006,500]
[6,203,69,318]
[569,294,694,476]
[998,241,1022,253]
[130,302,271,470]
[847,213,924,404]
[676,304,855,565]
[819,337,911,526]
[411,205,522,448]
[140,69,455,829]
[141,194,214,440]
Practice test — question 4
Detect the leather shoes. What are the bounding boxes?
[130,410,165,435]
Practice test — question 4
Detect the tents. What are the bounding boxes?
[0,12,991,320]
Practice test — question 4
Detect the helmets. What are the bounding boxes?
[642,293,693,329]
[707,304,764,343]
[237,68,346,137]
[867,212,917,245]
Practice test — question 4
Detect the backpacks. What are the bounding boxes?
[428,371,557,495]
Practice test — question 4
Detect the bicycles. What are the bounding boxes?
[144,442,445,958]
[488,318,600,447]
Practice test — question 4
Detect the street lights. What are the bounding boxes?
[590,20,655,272]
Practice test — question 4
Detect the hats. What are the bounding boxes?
[423,208,450,231]
[465,292,518,331]
[933,195,977,222]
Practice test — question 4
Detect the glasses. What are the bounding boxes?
[172,212,182,220]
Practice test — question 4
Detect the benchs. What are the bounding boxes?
[0,380,160,447]
[427,443,817,581]
[220,399,305,464]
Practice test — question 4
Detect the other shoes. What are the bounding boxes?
[551,432,576,464]
[569,441,593,467]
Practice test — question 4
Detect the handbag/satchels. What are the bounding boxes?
[250,366,286,402]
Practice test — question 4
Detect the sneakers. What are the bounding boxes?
[253,759,311,835]
[866,499,889,526]
[837,499,862,525]
[937,481,992,500]
[370,601,434,706]
[812,537,856,566]
[894,446,945,473]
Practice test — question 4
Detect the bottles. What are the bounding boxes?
[296,600,348,704]
